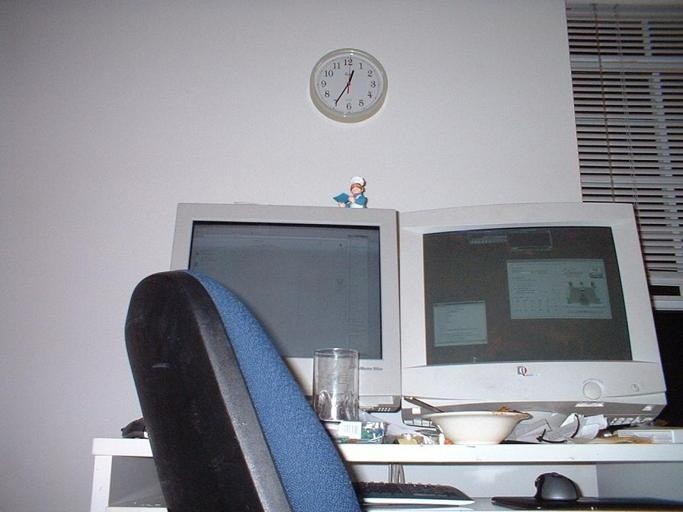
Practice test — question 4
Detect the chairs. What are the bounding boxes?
[126,271,362,512]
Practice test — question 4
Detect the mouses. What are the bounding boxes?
[121,422,149,439]
[534,472,581,500]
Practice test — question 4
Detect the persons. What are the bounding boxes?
[331,176,368,210]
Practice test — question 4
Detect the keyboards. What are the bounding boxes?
[352,481,475,506]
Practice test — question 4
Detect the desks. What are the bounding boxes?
[87,427,683,512]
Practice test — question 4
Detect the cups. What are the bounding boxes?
[310,346,360,422]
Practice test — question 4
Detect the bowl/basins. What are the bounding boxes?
[421,409,530,444]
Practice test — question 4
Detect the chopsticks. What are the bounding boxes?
[403,393,444,414]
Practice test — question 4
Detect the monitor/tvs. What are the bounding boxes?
[170,202,402,413]
[397,202,668,443]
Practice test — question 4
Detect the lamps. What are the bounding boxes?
[310,47,388,124]
